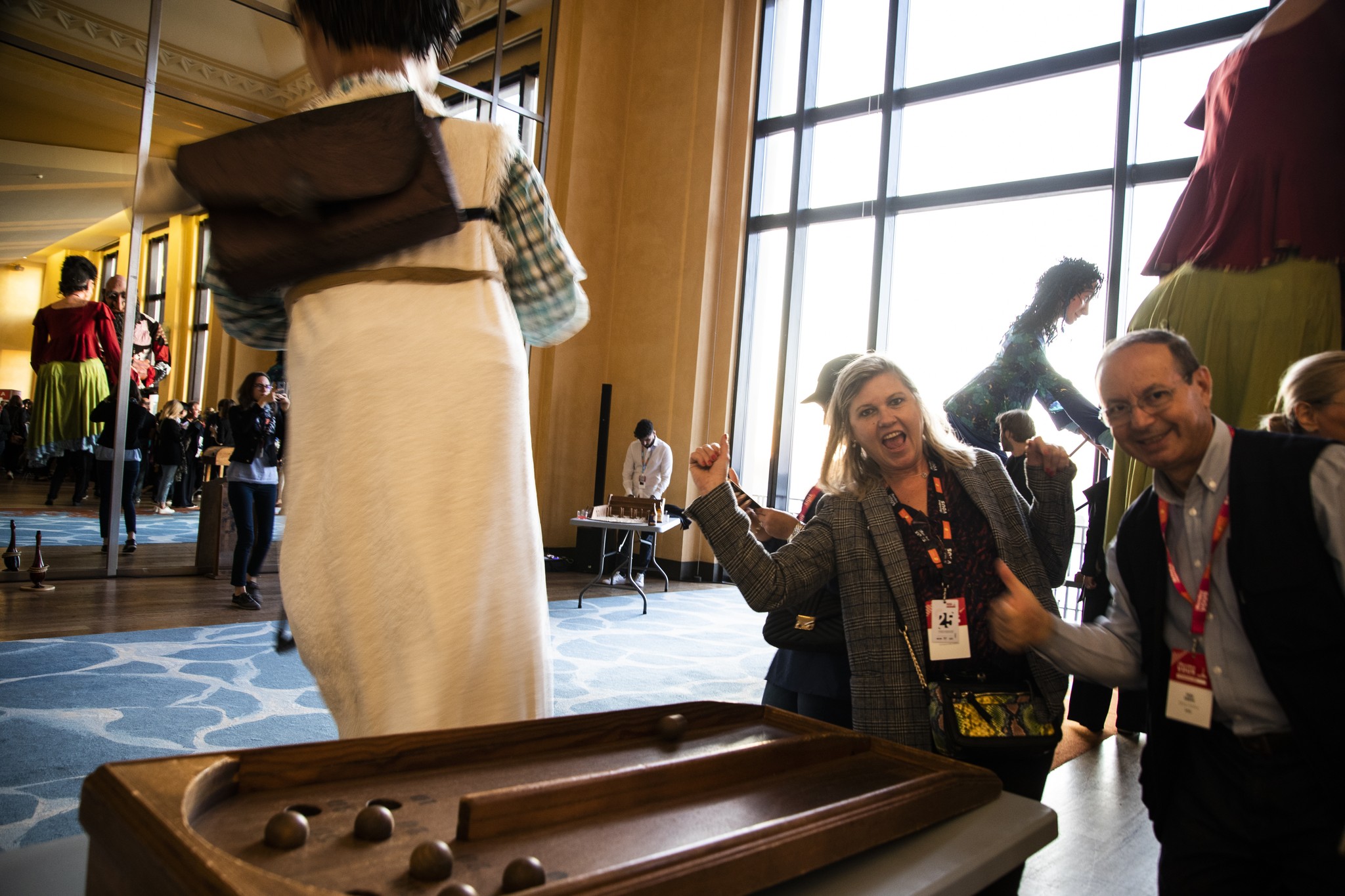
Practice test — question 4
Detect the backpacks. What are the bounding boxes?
[174,90,464,299]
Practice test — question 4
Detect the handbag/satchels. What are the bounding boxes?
[762,588,846,652]
[927,671,1063,759]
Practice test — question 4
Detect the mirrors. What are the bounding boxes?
[0,0,553,572]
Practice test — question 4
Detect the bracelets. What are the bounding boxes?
[754,522,760,536]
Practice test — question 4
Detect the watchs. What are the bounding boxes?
[787,520,805,544]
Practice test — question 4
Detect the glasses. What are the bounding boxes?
[1097,373,1192,428]
[252,383,273,392]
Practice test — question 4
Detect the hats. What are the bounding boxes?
[799,353,864,404]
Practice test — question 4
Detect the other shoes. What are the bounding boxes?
[73,502,80,506]
[177,503,194,508]
[45,499,54,505]
[192,503,197,508]
[133,498,141,503]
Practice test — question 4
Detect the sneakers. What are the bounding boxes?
[246,580,261,604]
[7,471,13,480]
[151,500,160,505]
[231,592,261,610]
[635,572,644,587]
[153,506,159,513]
[122,539,137,553]
[159,506,175,514]
[101,545,108,554]
[602,571,626,585]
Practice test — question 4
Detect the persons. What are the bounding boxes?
[683,351,1078,896]
[89,379,158,553]
[216,371,290,611]
[99,276,171,502]
[1067,476,1144,745]
[727,351,876,730]
[1254,352,1345,442]
[941,255,1114,461]
[149,397,236,514]
[988,334,1345,896]
[0,394,34,480]
[1099,1,1345,549]
[993,410,1036,503]
[601,418,673,587]
[197,1,591,732]
[30,254,150,504]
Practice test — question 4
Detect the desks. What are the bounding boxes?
[569,518,681,614]
[743,793,1059,895]
